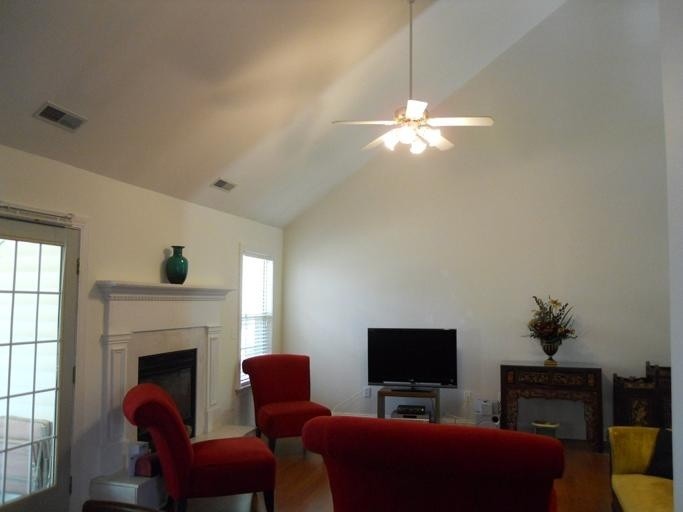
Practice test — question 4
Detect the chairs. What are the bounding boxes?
[123,383,276,512]
[609,425,673,512]
[242,354,331,454]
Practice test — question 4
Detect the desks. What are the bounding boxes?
[501,361,603,452]
[378,388,440,423]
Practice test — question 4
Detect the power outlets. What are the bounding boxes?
[363,386,371,397]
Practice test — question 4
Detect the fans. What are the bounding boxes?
[333,0,494,151]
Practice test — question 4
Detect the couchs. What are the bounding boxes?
[302,416,565,512]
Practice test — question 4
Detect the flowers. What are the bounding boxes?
[518,295,587,344]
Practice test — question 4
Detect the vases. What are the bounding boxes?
[542,337,558,365]
[166,246,188,284]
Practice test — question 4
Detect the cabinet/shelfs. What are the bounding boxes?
[646,362,671,428]
[614,374,659,427]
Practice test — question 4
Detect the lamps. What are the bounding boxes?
[384,129,441,154]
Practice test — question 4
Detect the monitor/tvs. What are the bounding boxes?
[368,328,458,392]
